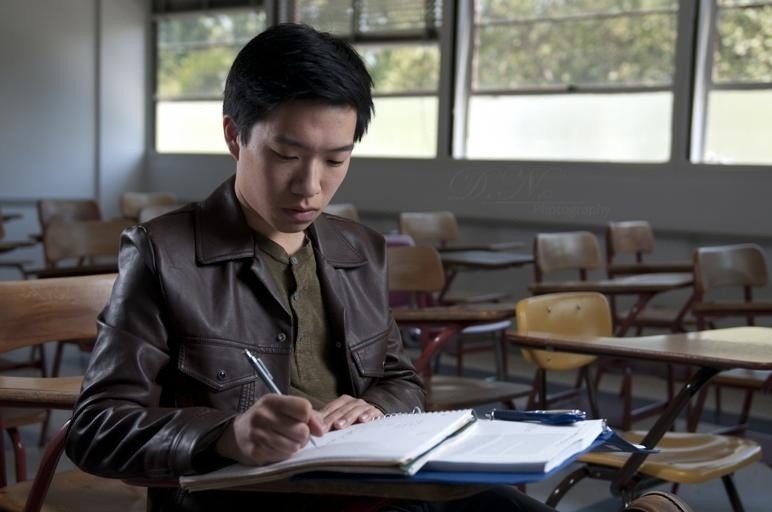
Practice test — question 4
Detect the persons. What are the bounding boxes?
[65,21,691,512]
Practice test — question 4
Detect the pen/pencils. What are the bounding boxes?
[242,348,320,449]
[485,408,588,425]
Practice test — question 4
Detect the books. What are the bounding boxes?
[178,404,476,494]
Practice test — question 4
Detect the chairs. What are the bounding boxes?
[0,274,148,511]
[504,292,772,510]
